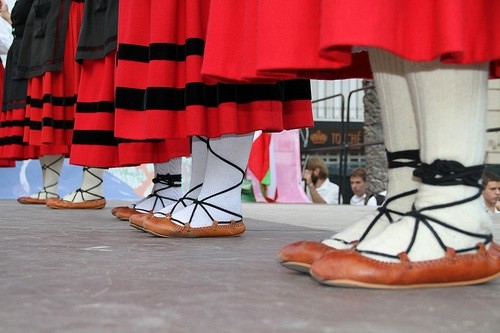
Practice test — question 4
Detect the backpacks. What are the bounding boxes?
[376,193,385,205]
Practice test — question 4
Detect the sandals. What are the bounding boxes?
[47,198,106,209]
[116,210,139,219]
[17,196,59,204]
[277,240,340,272]
[111,207,129,215]
[143,217,246,238]
[129,213,155,229]
[309,243,499,288]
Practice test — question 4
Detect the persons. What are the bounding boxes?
[0,0,500,333]
